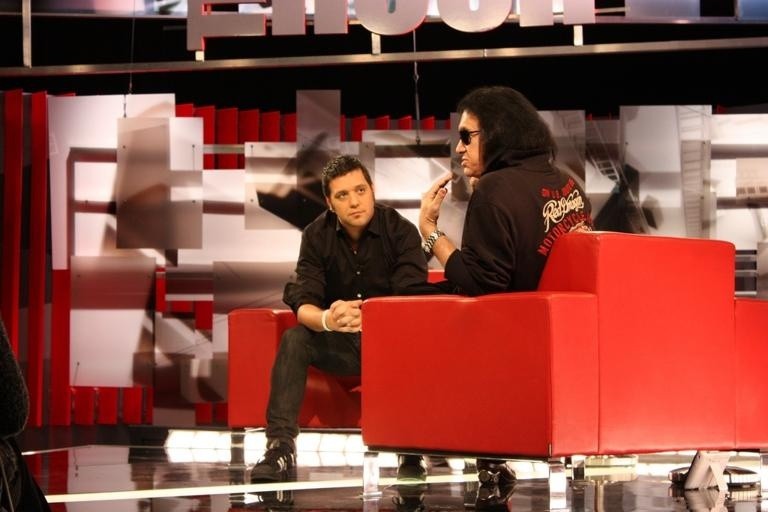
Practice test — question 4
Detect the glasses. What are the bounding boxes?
[457,127,480,145]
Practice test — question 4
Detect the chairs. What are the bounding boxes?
[361,229,768,496]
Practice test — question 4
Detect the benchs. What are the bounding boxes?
[227,269,445,471]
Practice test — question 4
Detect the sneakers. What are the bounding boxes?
[250,440,296,479]
[476,461,516,484]
[397,454,427,481]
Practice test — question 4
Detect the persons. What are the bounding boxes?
[418,87,598,485]
[475,485,521,511]
[251,155,431,485]
[251,486,431,511]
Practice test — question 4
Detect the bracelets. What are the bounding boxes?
[422,228,446,255]
[321,309,332,332]
